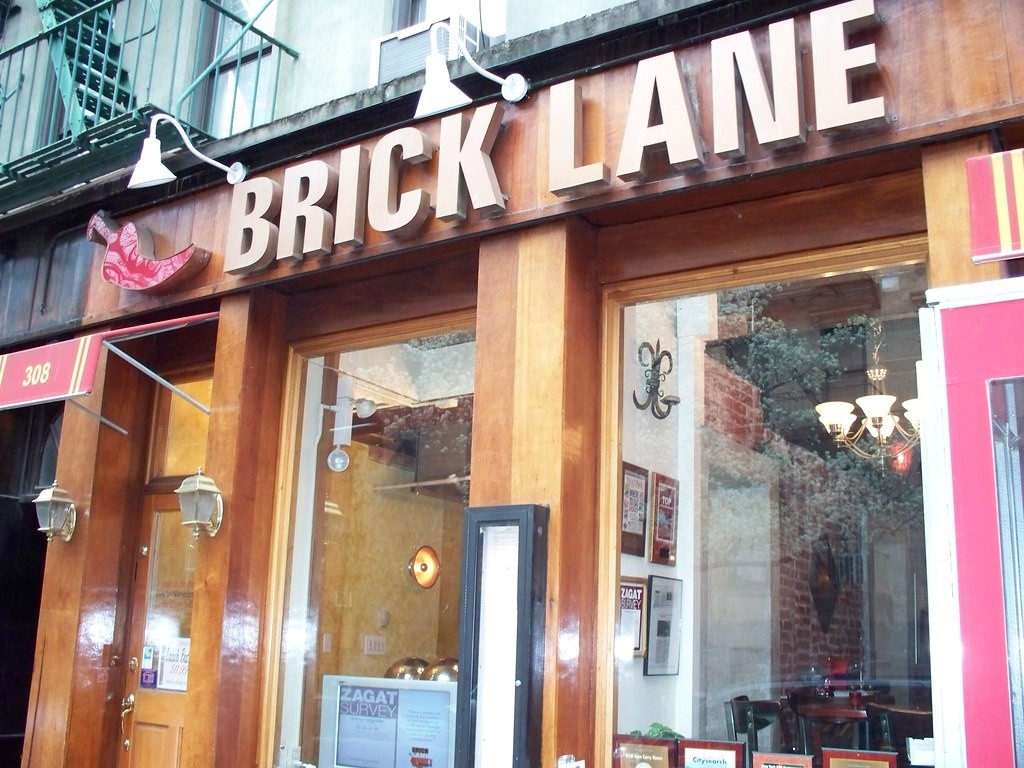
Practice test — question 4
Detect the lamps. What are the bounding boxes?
[414,22,528,120]
[814,320,922,479]
[175,465,225,542]
[128,113,248,188]
[31,479,77,546]
[316,375,376,472]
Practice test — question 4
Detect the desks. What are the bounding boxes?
[797,703,870,755]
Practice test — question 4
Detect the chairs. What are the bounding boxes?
[866,702,934,768]
[724,695,781,768]
